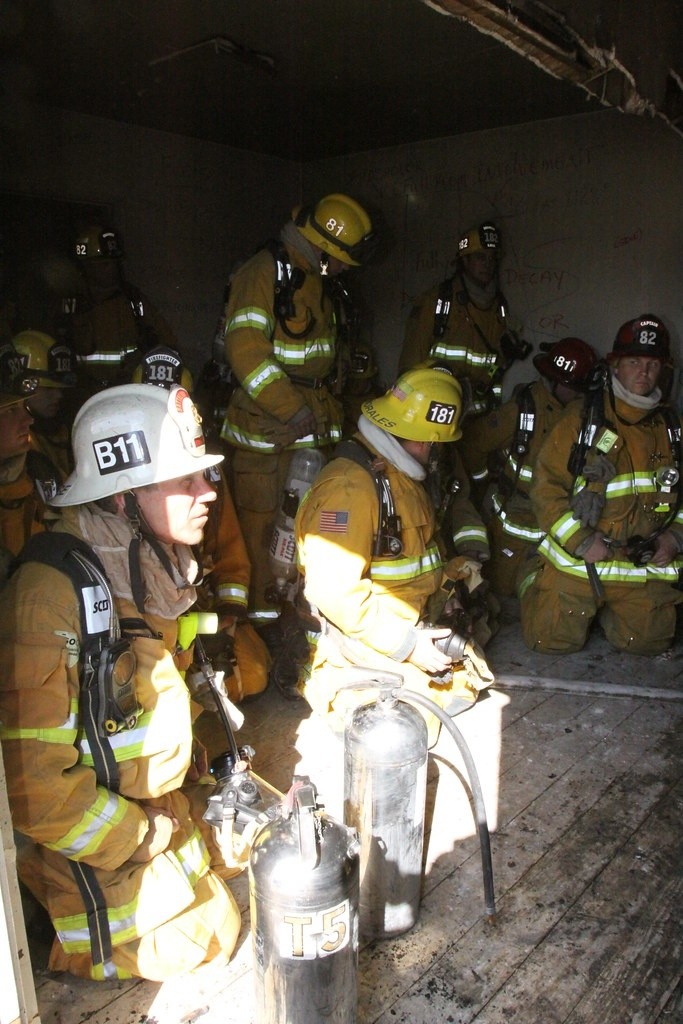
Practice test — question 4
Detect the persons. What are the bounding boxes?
[0,194,513,712]
[0,384,265,982]
[291,368,491,750]
[463,339,598,599]
[518,317,683,655]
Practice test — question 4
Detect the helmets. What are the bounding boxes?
[612,314,670,363]
[48,383,225,508]
[296,193,373,270]
[458,221,502,254]
[360,367,464,442]
[533,338,599,391]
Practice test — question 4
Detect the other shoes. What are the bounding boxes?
[279,683,301,701]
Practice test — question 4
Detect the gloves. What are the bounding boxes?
[571,457,615,528]
[257,406,327,452]
